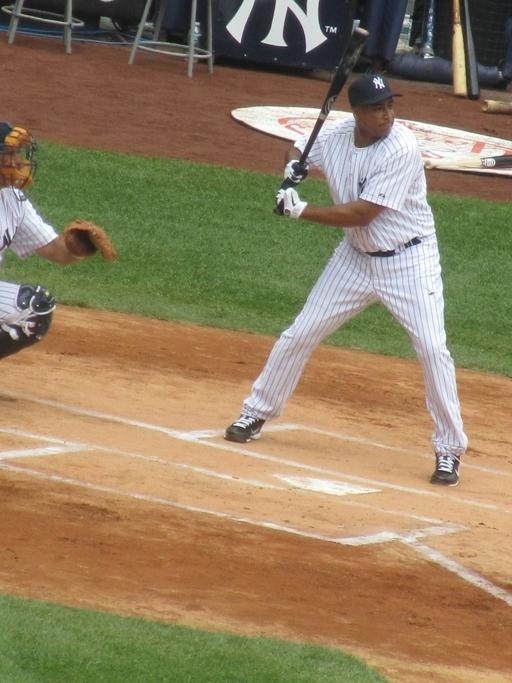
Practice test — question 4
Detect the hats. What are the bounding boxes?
[348,75,403,105]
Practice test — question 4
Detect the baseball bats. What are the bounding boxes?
[273,28,370,215]
[482,99,512,114]
[453,0,479,101]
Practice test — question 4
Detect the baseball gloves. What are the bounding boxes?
[64,218,116,262]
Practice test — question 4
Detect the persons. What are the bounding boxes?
[217,69,471,487]
[0,118,122,366]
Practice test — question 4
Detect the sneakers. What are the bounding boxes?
[431,454,460,487]
[224,414,265,442]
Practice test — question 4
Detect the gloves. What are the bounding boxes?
[275,187,309,219]
[284,160,310,184]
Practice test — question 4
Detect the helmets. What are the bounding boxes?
[0,122,38,200]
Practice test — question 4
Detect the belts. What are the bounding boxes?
[365,238,421,257]
